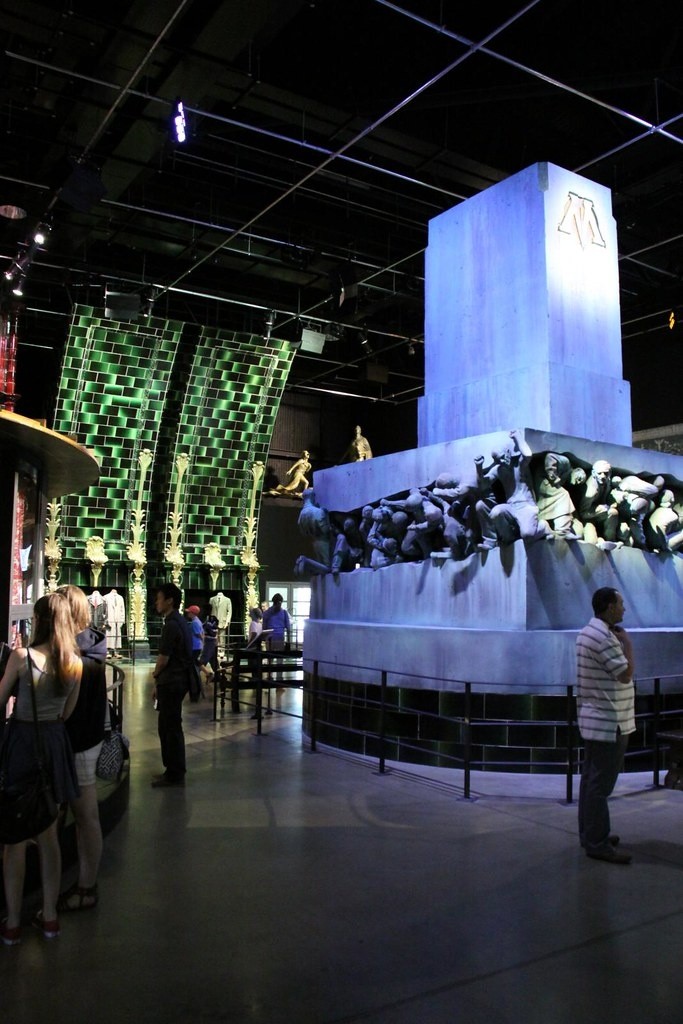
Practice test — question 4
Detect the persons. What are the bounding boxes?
[276,450,312,492]
[103,589,125,659]
[87,591,108,635]
[261,601,269,613]
[198,603,222,682]
[576,587,637,865]
[185,605,215,685]
[0,593,83,945]
[340,425,373,465]
[248,609,263,697]
[151,583,193,787]
[294,428,683,578]
[263,593,290,691]
[210,592,232,656]
[53,584,107,912]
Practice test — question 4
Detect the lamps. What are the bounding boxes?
[358,331,366,344]
[4,211,52,295]
[143,303,151,317]
[264,311,273,324]
[146,287,156,300]
[263,326,270,339]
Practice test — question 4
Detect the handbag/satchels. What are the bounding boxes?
[0,767,59,844]
[95,732,130,781]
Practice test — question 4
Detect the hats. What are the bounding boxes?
[185,605,201,614]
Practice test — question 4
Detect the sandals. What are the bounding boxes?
[57,888,98,912]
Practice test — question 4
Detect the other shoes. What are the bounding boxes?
[206,672,214,685]
[581,835,620,847]
[31,910,62,937]
[151,772,185,788]
[587,849,632,864]
[0,918,22,945]
